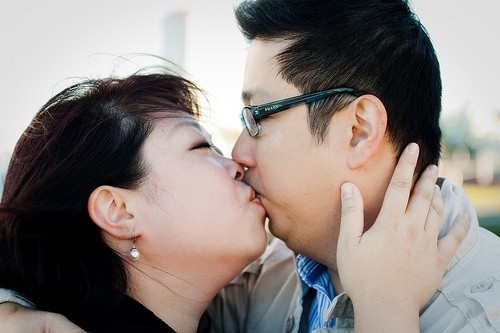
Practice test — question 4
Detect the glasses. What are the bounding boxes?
[238,85,367,139]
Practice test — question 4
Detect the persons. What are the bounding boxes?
[0,0,499,333]
